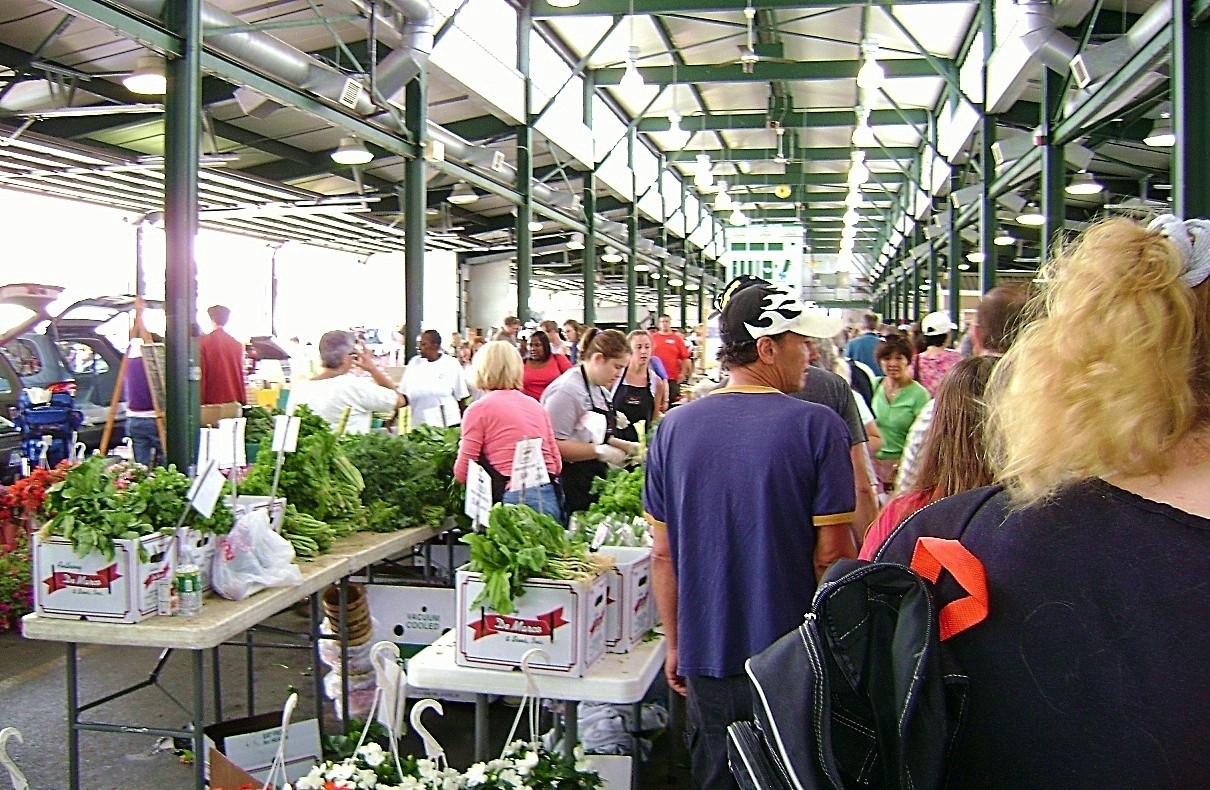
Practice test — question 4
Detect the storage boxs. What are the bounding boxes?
[34,492,287,623]
[576,755,633,790]
[188,711,323,790]
[333,544,664,703]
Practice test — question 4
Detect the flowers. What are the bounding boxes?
[0,459,254,636]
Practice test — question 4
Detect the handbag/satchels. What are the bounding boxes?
[208,507,301,601]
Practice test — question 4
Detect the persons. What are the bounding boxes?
[280,213,1210,790]
[197,305,247,407]
[123,331,164,472]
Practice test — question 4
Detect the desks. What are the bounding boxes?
[407,620,669,790]
[21,517,458,790]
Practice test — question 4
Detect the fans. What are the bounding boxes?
[747,122,811,167]
[715,0,796,69]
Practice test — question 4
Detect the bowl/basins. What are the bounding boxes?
[322,583,371,647]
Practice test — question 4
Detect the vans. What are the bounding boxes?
[0,282,164,486]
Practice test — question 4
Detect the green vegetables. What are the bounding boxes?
[457,501,613,617]
[40,454,238,565]
[228,404,469,556]
[575,467,651,551]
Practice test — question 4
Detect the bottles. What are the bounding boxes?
[175,543,204,615]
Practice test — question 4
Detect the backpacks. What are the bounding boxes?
[728,479,1007,790]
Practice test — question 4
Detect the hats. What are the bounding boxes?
[709,274,772,320]
[921,313,959,335]
[719,286,843,347]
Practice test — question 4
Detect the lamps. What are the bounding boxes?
[122,49,167,95]
[617,0,746,227]
[854,37,884,145]
[1144,112,1175,146]
[1065,170,1102,195]
[993,227,1014,245]
[1016,194,1042,225]
[331,138,374,165]
[446,180,478,203]
